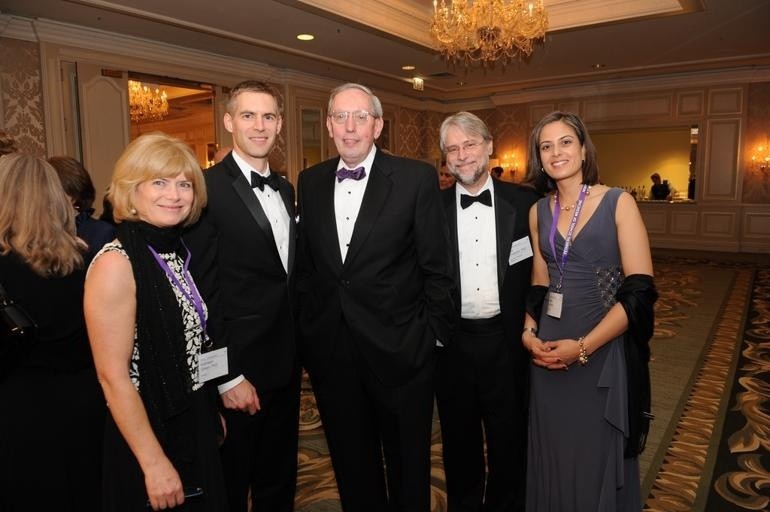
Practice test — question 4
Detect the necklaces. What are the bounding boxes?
[552,184,594,211]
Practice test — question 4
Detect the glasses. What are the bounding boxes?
[443,137,487,155]
[328,111,379,123]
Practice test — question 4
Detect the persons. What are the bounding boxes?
[436,159,456,191]
[0,145,95,510]
[431,114,550,509]
[291,84,463,511]
[79,132,233,511]
[38,153,121,249]
[192,80,304,508]
[514,107,660,508]
[492,165,505,178]
[650,173,673,202]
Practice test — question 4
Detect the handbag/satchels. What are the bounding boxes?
[0,294,43,385]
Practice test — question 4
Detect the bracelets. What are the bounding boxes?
[521,327,537,336]
[576,333,587,368]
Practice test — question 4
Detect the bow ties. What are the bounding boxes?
[249,169,282,193]
[459,187,494,210]
[333,166,367,183]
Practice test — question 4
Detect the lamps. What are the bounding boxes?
[501,150,523,181]
[424,0,549,69]
[127,76,172,124]
[749,145,770,188]
